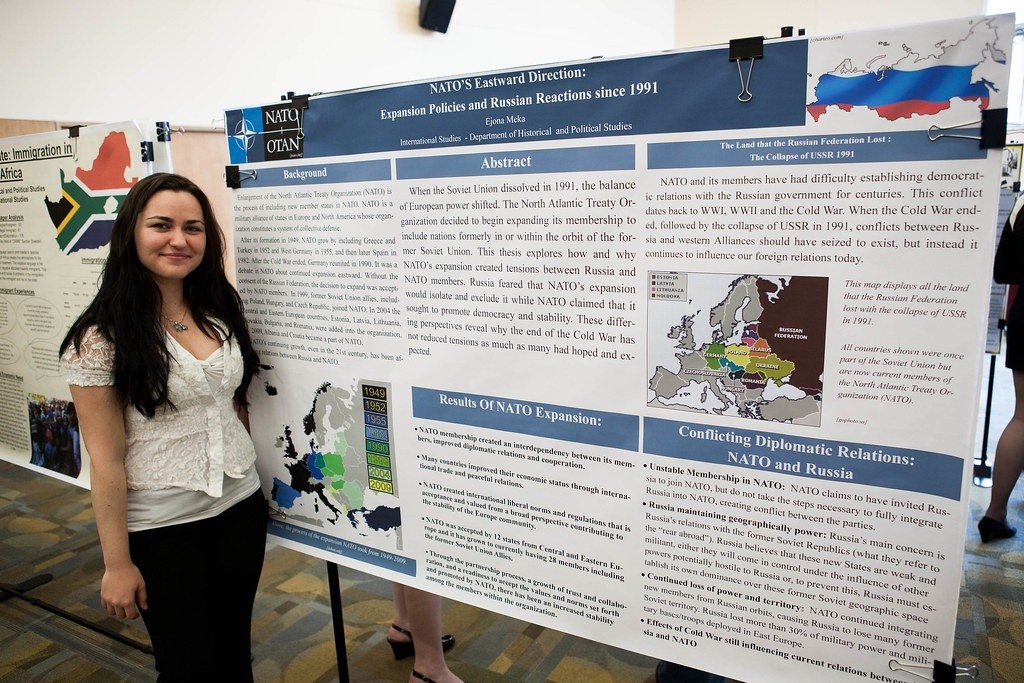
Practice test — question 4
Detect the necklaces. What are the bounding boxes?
[162,307,188,333]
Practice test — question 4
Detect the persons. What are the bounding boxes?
[28,398,81,478]
[655,660,727,683]
[977,194,1024,544]
[387,581,464,683]
[59,173,269,683]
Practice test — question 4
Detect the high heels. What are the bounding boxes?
[408,669,436,683]
[978,516,1017,543]
[387,624,455,660]
